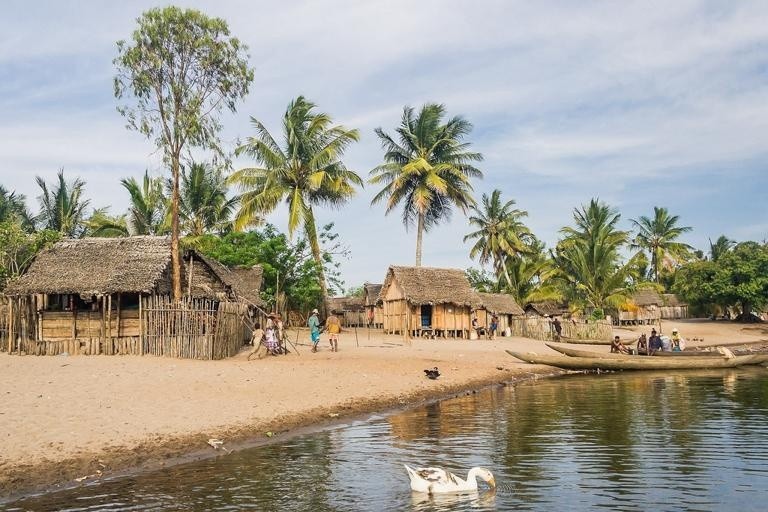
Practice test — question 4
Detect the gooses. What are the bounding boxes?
[403,463,495,494]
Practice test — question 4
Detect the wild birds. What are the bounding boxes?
[424,367,441,379]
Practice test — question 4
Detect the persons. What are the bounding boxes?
[610,336,630,353]
[265,312,284,356]
[472,318,481,338]
[307,309,324,353]
[489,316,497,339]
[367,307,375,328]
[552,318,561,336]
[320,310,342,352]
[709,307,731,321]
[247,323,265,361]
[636,327,686,356]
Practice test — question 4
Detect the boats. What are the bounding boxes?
[503,342,767,370]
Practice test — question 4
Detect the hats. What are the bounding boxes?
[269,313,276,317]
[312,309,319,314]
